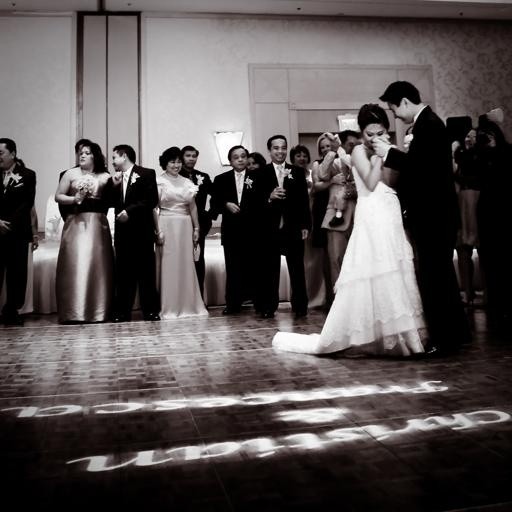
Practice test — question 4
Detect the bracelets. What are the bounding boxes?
[33,235,39,238]
[154,229,161,235]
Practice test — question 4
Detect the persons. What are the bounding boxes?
[181,145,212,302]
[212,146,254,316]
[273,103,427,357]
[312,131,333,246]
[58,139,92,222]
[0,137,36,325]
[454,127,481,305]
[290,144,326,308]
[246,152,266,170]
[372,80,461,355]
[319,130,358,284]
[104,144,160,323]
[256,135,310,318]
[155,146,209,318]
[18,157,39,314]
[54,143,113,324]
[329,146,357,227]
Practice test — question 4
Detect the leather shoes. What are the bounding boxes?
[261,312,274,318]
[420,343,455,358]
[222,307,236,314]
[113,315,128,322]
[144,313,160,321]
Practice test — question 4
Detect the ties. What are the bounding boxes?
[276,164,283,188]
[121,172,128,201]
[235,173,242,204]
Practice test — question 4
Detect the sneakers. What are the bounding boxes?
[328,216,344,226]
